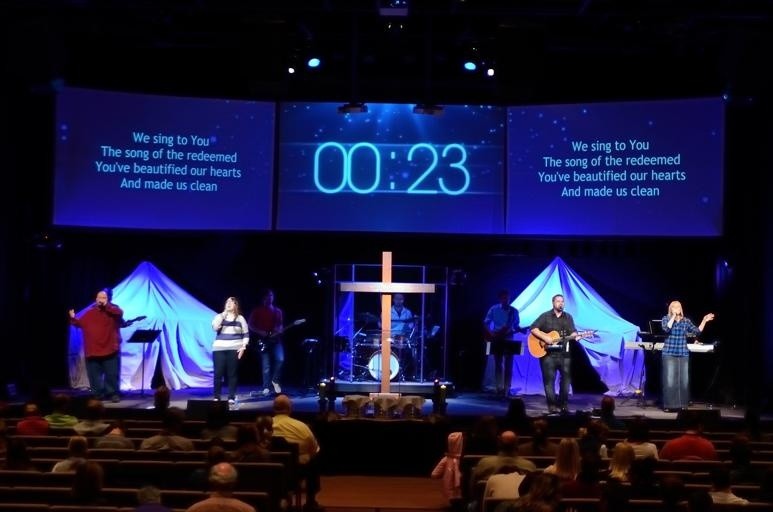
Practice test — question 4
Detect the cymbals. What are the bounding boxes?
[358,312,379,323]
[403,314,431,323]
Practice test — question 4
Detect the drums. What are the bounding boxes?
[365,329,382,348]
[364,350,403,384]
[358,332,366,344]
[393,335,409,350]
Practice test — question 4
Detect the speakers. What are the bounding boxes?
[188,400,228,419]
[48,391,95,417]
[676,410,721,427]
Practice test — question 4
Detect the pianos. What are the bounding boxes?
[624,342,716,354]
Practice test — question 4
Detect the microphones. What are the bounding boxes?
[560,307,563,310]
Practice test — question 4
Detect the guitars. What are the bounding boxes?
[525,329,603,359]
[120,316,146,329]
[459,326,531,358]
[254,318,307,353]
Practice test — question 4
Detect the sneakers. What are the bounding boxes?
[228,399,235,404]
[214,399,219,401]
[272,381,281,393]
[262,388,269,395]
[111,394,120,402]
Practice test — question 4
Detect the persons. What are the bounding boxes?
[271,395,321,509]
[658,299,716,413]
[52,436,115,507]
[209,296,251,407]
[462,394,760,511]
[140,385,194,451]
[65,287,125,403]
[136,486,174,511]
[246,287,290,397]
[527,293,582,415]
[14,394,135,448]
[480,288,529,404]
[187,463,255,511]
[187,404,295,491]
[376,290,422,380]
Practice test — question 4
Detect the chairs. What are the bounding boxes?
[2,402,771,512]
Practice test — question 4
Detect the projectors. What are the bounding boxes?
[343,104,368,112]
[413,105,443,114]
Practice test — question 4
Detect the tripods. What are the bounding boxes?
[129,341,153,399]
[338,326,429,382]
